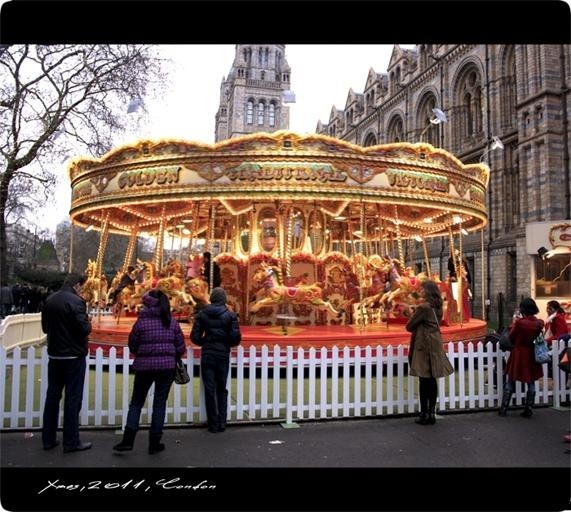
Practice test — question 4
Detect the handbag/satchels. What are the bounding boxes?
[534,319,551,363]
[174,359,191,384]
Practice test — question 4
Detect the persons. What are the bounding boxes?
[0,283,12,318]
[12,282,21,314]
[406,280,454,424]
[543,300,567,346]
[497,298,544,417]
[41,273,92,453]
[112,289,187,454]
[202,252,221,287]
[42,287,52,302]
[447,249,474,300]
[106,266,136,307]
[21,282,31,313]
[34,286,44,313]
[190,286,241,432]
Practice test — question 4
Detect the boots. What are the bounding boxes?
[520,390,536,419]
[148,427,165,455]
[498,392,512,417]
[111,428,136,452]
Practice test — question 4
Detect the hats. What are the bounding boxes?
[141,291,160,307]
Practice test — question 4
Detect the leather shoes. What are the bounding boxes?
[42,437,60,450]
[63,440,93,453]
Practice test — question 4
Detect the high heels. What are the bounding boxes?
[430,407,436,425]
[414,413,430,425]
